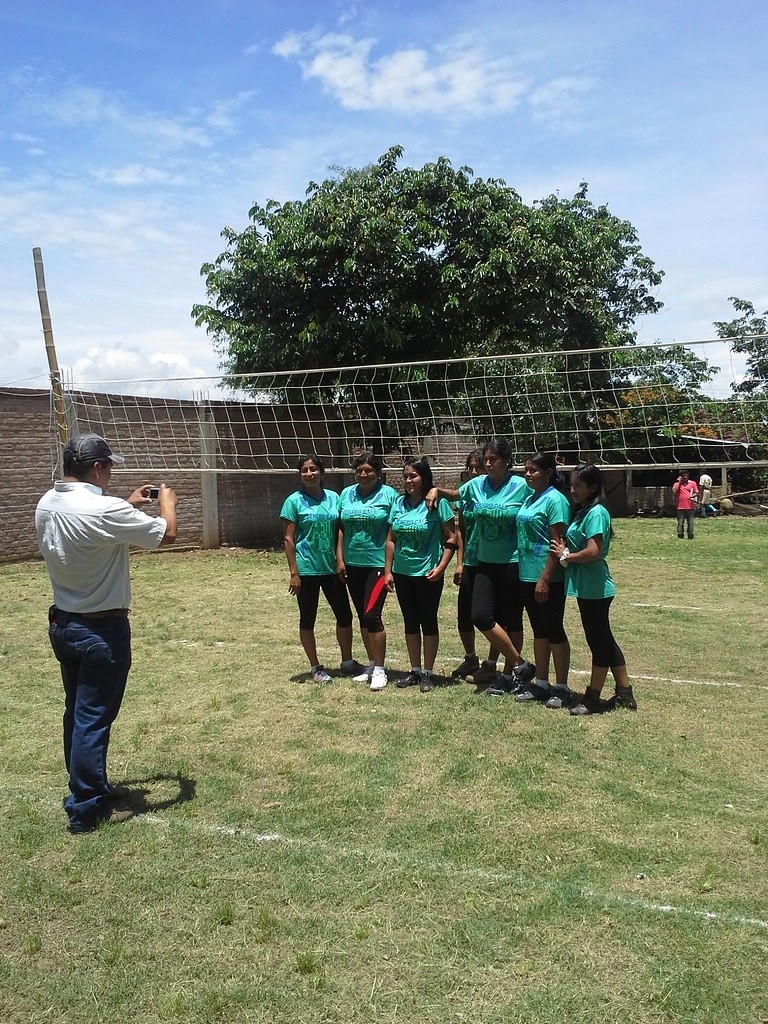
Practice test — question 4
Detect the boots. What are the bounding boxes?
[603,686,637,710]
[571,686,600,715]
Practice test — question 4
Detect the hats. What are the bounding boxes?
[63,433,125,464]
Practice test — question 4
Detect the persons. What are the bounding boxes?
[672,470,699,540]
[336,452,399,691]
[384,459,456,692]
[279,453,367,682]
[698,468,718,518]
[514,452,573,708]
[35,433,180,833]
[548,463,637,715]
[425,436,536,697]
[452,448,501,683]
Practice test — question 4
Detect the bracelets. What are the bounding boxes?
[559,548,569,567]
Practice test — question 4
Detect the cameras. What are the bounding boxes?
[148,488,161,501]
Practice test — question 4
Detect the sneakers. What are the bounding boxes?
[353,664,374,681]
[509,661,536,694]
[546,687,574,708]
[488,675,513,696]
[396,671,419,688]
[451,656,479,675]
[309,663,333,683]
[369,666,388,690]
[515,683,553,702]
[420,673,435,692]
[340,660,366,677]
[465,660,496,684]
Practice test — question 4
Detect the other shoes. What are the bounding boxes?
[67,809,135,832]
[112,786,134,802]
